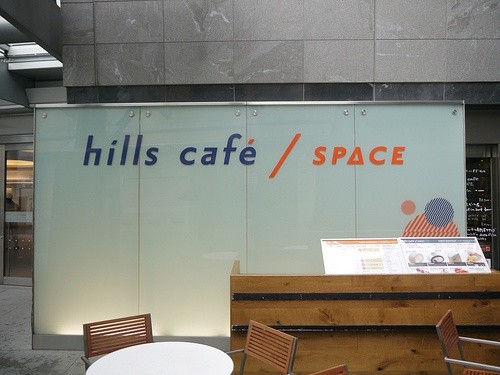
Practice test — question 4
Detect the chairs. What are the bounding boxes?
[80,313,154,372]
[226,318,300,375]
[434,308,500,375]
[309,364,352,375]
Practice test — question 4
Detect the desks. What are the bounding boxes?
[86,341,235,375]
